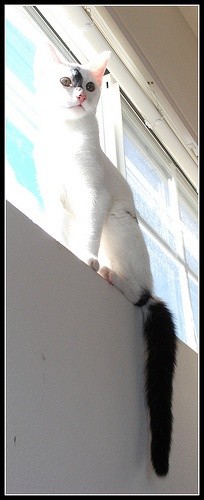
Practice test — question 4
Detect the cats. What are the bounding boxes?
[28,33,174,477]
